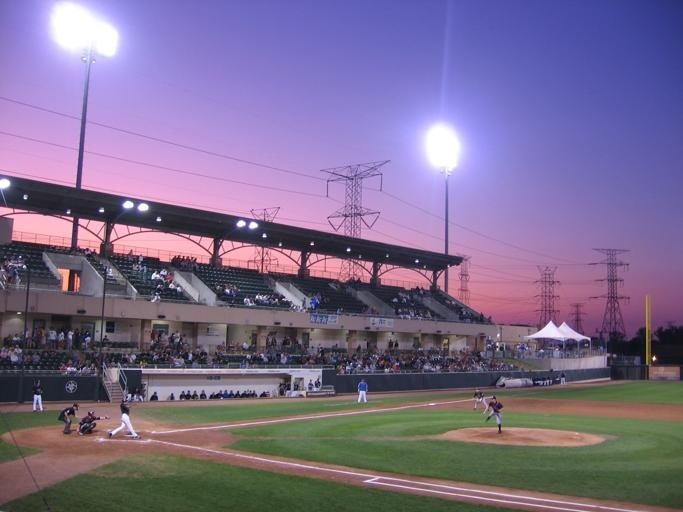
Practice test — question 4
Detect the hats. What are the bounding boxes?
[490,395,497,399]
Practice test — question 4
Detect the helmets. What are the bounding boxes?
[71,398,131,417]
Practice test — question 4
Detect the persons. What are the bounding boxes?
[57,402,80,435]
[109,398,142,438]
[485,395,503,434]
[78,408,110,435]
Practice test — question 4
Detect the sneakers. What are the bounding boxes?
[62,428,144,439]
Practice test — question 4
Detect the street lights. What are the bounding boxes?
[428,127,455,296]
[50,3,120,250]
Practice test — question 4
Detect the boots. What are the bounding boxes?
[484,416,502,434]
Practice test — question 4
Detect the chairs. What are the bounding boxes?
[368,285,474,319]
[2,241,57,285]
[90,259,118,285]
[58,248,89,257]
[108,353,311,366]
[271,272,376,316]
[110,254,192,303]
[3,351,74,371]
[175,258,292,309]
